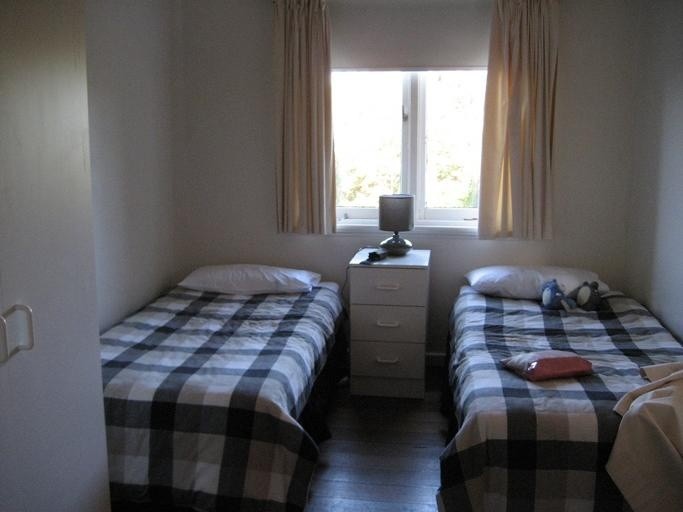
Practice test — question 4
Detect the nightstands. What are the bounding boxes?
[349,247,432,399]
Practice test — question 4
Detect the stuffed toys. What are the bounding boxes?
[540,278,602,313]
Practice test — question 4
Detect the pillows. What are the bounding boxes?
[464,265,609,299]
[177,262,321,294]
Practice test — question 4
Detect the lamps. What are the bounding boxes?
[378,195,415,254]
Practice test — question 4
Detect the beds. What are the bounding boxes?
[100,281,343,512]
[437,284,682,511]
